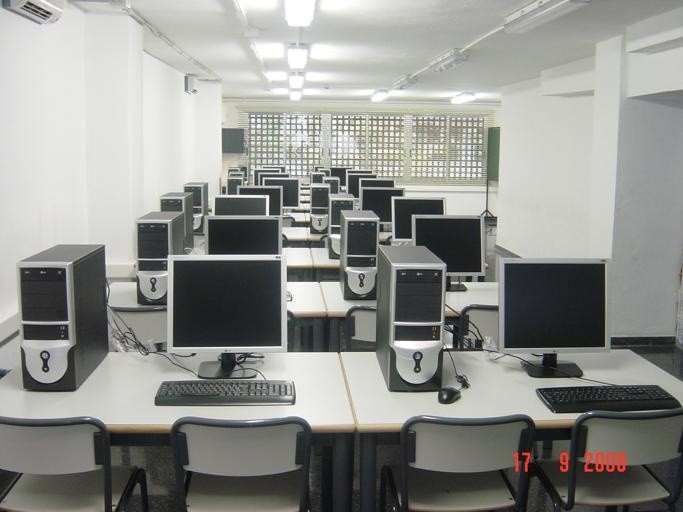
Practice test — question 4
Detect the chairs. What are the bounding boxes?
[0,173,681,512]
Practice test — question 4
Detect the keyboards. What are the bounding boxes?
[535,384,681,413]
[154,380,296,405]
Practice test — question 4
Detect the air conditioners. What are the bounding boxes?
[1,1,67,25]
[182,73,198,94]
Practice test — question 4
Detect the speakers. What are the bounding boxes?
[222,128,243,153]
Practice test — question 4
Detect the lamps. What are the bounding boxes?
[287,44,309,69]
[288,72,305,90]
[284,0,316,27]
[389,74,420,91]
[291,91,302,101]
[370,89,388,103]
[502,0,589,36]
[428,48,469,73]
[451,92,476,105]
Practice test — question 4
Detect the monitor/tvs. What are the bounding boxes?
[166,254,289,355]
[204,216,284,255]
[411,214,486,278]
[330,168,395,199]
[360,187,406,225]
[254,166,301,210]
[499,258,612,355]
[391,196,446,242]
[213,195,271,217]
[237,185,284,217]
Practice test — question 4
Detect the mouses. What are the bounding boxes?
[438,387,461,403]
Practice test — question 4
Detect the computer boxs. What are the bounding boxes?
[136,211,183,306]
[326,195,356,260]
[160,192,193,253]
[16,244,106,391]
[310,183,331,235]
[226,168,245,196]
[375,245,445,392]
[311,166,341,195]
[184,182,209,235]
[340,210,381,301]
